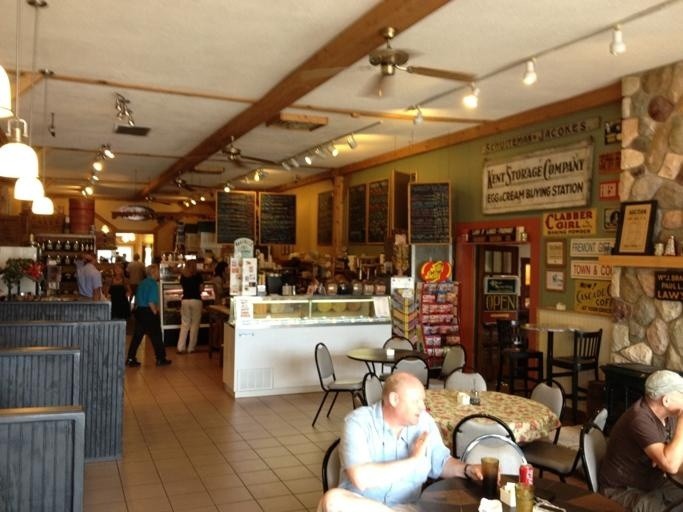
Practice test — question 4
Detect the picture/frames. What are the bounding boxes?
[545,240,567,292]
[601,200,657,255]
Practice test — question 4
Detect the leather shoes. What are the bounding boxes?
[125,358,141,367]
[156,359,171,366]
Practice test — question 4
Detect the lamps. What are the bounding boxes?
[0,0,54,215]
[90,176,99,185]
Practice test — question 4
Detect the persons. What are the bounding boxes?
[598,368,683,512]
[106,262,133,332]
[175,259,205,355]
[125,264,174,368]
[77,247,218,301]
[316,372,502,512]
[213,261,234,298]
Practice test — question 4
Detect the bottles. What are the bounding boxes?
[653,234,674,256]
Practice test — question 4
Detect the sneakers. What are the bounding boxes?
[176,349,193,353]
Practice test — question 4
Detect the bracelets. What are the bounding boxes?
[463,463,473,479]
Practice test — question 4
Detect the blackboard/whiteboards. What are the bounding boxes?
[348,183,366,243]
[215,189,256,246]
[317,191,333,245]
[407,179,452,246]
[258,191,299,246]
[367,179,388,243]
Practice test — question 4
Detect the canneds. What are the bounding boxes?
[519,462,535,486]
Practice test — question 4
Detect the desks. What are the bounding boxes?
[207,305,229,370]
[599,363,683,438]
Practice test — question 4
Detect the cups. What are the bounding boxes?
[481,457,499,498]
[515,483,535,512]
[470,379,481,404]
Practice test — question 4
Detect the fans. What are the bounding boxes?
[132,170,206,205]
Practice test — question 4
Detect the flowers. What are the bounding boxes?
[19,257,48,302]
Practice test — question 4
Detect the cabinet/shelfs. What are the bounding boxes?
[34,234,96,273]
[223,296,393,398]
[160,280,218,343]
